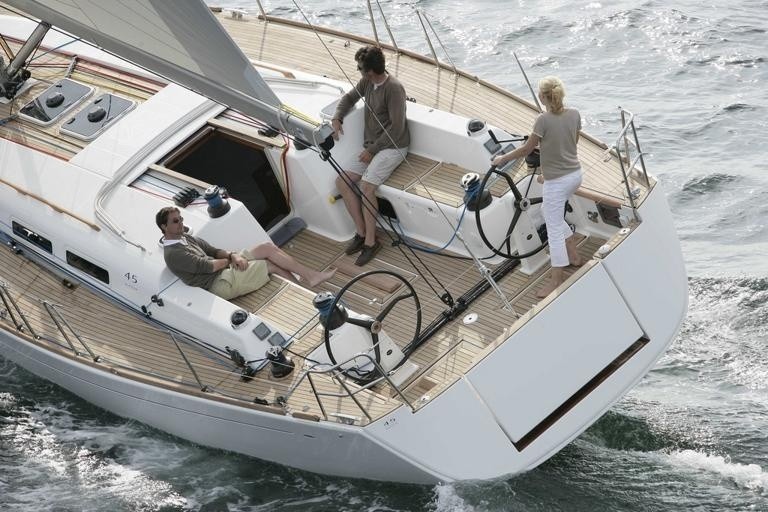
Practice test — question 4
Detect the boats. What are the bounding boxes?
[1,0,689,483]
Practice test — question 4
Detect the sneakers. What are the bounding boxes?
[355,241,382,266]
[346,233,365,254]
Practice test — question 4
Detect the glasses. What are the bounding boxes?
[172,217,184,223]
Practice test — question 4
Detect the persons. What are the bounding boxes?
[335,46,412,266]
[155,204,340,302]
[489,75,585,298]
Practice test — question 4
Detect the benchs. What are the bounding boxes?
[352,96,542,267]
[152,273,352,358]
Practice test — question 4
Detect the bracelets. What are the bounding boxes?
[227,250,237,261]
